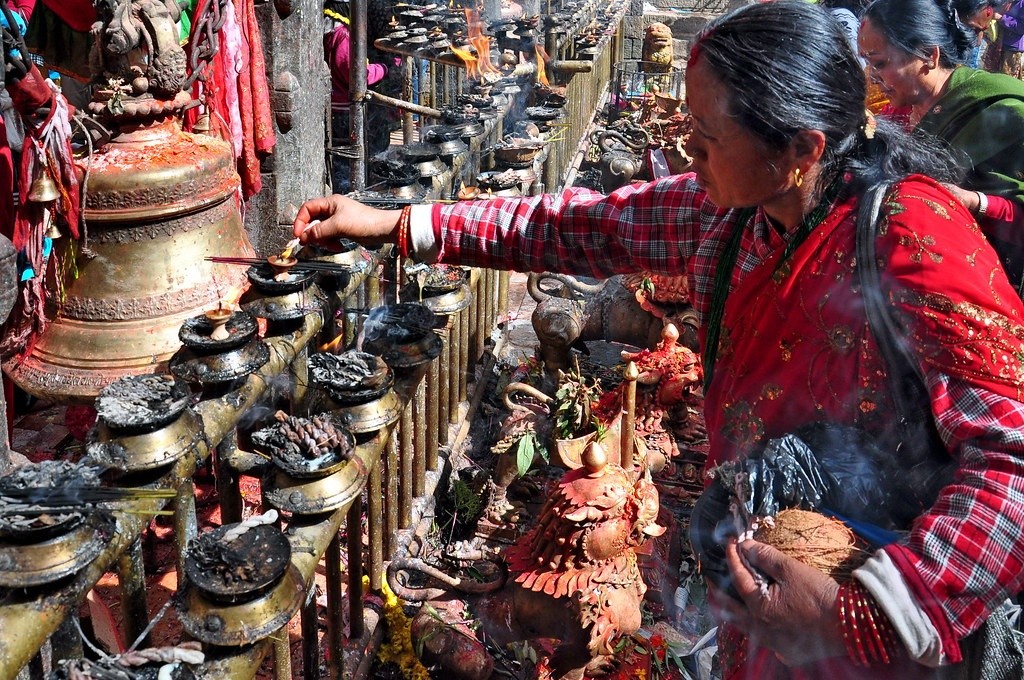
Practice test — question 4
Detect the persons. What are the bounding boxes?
[815,0,1023,300]
[291,0,1024,680]
[323,0,436,194]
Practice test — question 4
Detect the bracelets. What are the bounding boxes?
[399,206,411,258]
[837,578,910,670]
